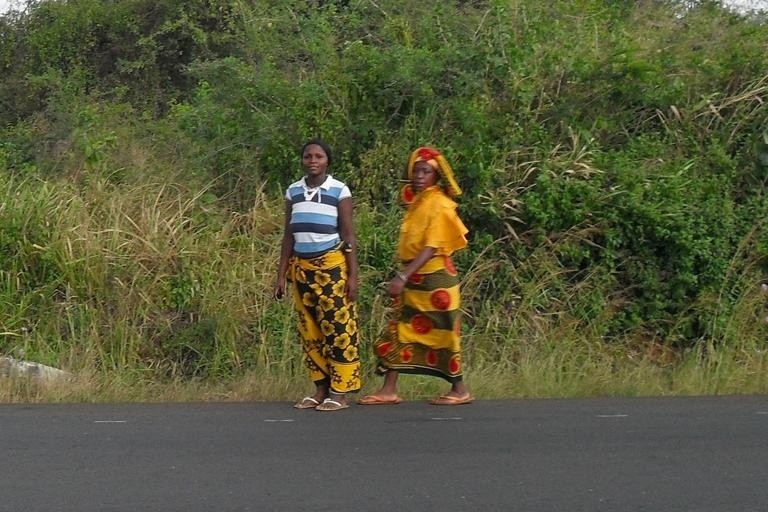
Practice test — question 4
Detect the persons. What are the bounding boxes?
[356,147,475,406]
[274,140,363,411]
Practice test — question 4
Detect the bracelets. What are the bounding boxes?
[399,272,407,282]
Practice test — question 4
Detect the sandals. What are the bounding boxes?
[431,392,473,405]
[356,395,401,405]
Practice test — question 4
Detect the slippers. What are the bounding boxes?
[293,396,350,411]
